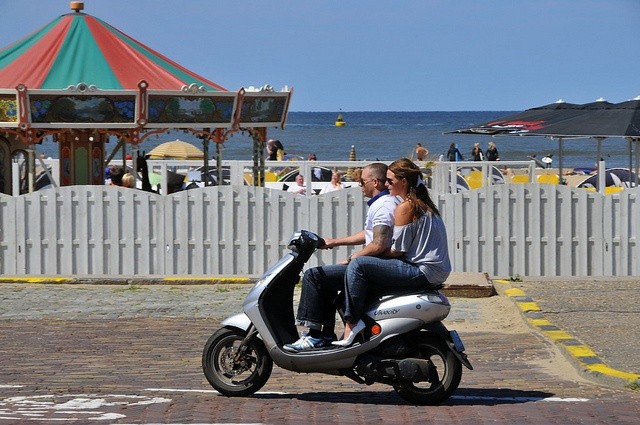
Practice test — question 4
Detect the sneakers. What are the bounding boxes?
[283,331,327,352]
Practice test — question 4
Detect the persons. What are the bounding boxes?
[110,165,125,186]
[501,166,507,175]
[330,158,451,347]
[416,143,428,161]
[287,174,316,196]
[266,140,278,161]
[121,173,135,188]
[439,153,444,161]
[310,154,316,161]
[472,143,484,160]
[447,143,464,161]
[546,153,554,168]
[319,172,345,194]
[126,152,133,160]
[274,141,284,161]
[282,163,401,353]
[486,142,498,160]
[40,153,45,159]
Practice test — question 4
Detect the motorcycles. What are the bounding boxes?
[202,230,472,405]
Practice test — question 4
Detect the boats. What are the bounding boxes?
[334,114,345,127]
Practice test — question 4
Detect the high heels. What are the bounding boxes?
[331,318,366,347]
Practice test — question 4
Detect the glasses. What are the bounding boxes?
[381,177,394,185]
[360,178,373,186]
